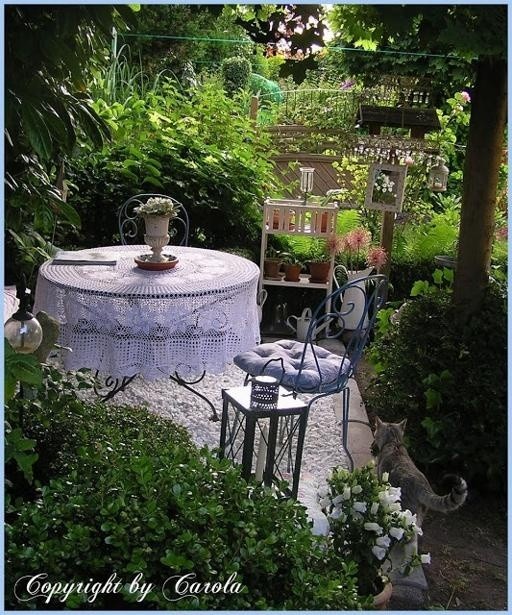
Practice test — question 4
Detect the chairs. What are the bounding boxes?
[118,193,189,246]
[232,273,389,473]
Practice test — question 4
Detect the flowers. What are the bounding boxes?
[134,197,183,218]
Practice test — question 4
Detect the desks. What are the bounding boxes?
[32,244,261,422]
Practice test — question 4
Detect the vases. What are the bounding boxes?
[143,213,169,236]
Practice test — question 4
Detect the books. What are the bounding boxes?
[51,250,117,265]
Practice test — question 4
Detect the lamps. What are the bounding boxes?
[4,274,43,356]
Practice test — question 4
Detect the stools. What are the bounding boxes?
[219,384,310,501]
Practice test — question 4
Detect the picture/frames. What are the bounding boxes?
[365,163,408,214]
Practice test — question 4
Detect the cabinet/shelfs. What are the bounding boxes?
[258,197,339,337]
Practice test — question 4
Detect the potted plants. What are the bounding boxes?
[264,244,330,284]
